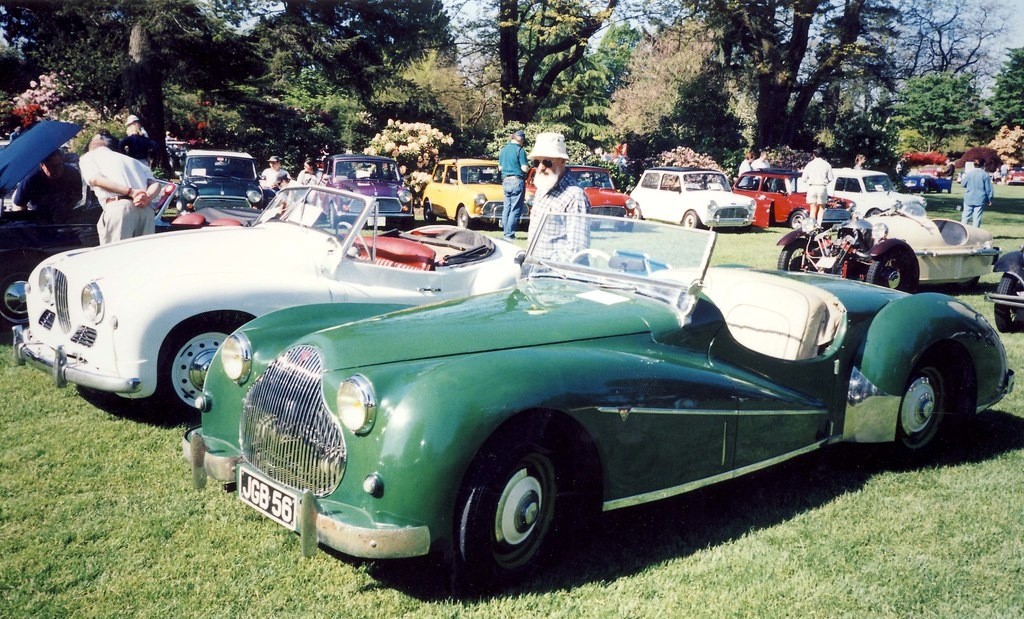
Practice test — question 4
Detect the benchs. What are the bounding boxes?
[651,265,827,361]
[344,232,434,274]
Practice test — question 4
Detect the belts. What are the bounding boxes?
[106,195,132,203]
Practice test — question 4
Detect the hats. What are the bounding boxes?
[516,131,525,140]
[267,155,279,162]
[275,173,287,181]
[527,132,570,160]
[125,115,140,125]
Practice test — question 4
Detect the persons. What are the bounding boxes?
[260,155,327,204]
[84,115,160,172]
[737,153,754,187]
[498,130,531,240]
[78,131,163,247]
[853,154,867,170]
[619,185,645,220]
[896,151,1010,185]
[9,125,23,145]
[960,156,993,228]
[752,150,772,184]
[526,133,592,269]
[802,148,834,227]
[11,148,83,213]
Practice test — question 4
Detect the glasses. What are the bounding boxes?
[532,159,555,168]
[278,180,283,185]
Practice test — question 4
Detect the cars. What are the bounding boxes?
[2,148,1024,588]
